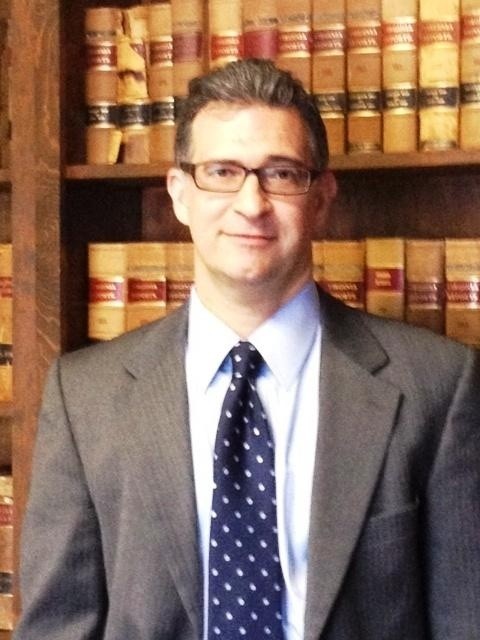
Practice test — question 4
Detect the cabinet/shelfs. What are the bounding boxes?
[0,0,479,640]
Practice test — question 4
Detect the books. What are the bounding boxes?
[88,239,480,344]
[72,1,479,167]
[0,243,14,403]
[0,475,13,631]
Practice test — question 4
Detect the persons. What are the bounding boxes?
[14,59,479,640]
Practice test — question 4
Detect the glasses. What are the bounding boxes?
[180,161,318,194]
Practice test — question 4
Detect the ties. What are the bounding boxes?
[208,342,286,640]
